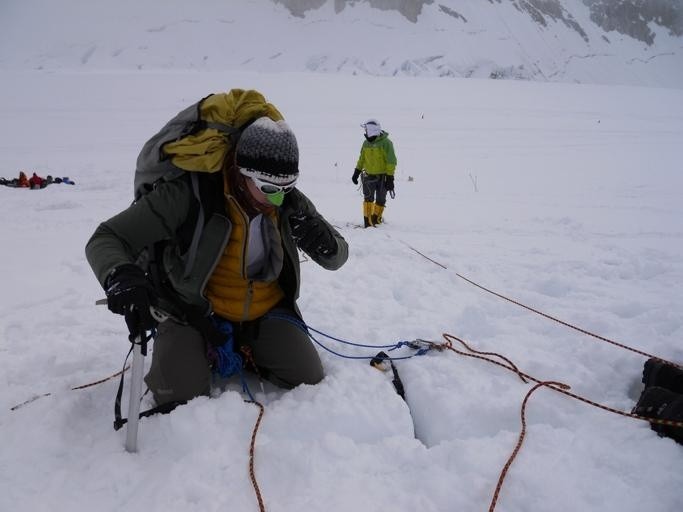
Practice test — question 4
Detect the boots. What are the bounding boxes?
[364,214,382,228]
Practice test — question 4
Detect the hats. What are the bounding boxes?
[235,115,299,184]
[360,119,381,138]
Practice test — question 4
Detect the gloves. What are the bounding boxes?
[385,174,394,190]
[288,210,337,260]
[105,263,172,337]
[351,168,362,184]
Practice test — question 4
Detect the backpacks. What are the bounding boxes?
[132,86,285,208]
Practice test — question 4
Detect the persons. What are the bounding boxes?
[78,89,349,415]
[349,120,396,228]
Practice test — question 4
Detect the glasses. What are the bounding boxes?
[251,176,294,195]
